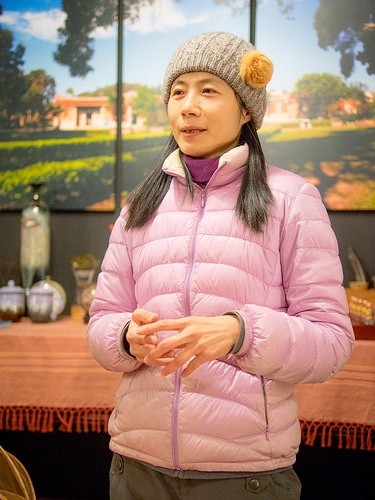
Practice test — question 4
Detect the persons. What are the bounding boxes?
[84,30,357,500]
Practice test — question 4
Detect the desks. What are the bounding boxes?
[0,303,375,446]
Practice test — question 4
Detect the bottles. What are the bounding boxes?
[17,180,52,285]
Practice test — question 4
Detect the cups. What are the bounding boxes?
[1,281,25,323]
[26,289,53,323]
[146,331,182,378]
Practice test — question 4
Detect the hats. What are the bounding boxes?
[162,31,273,130]
